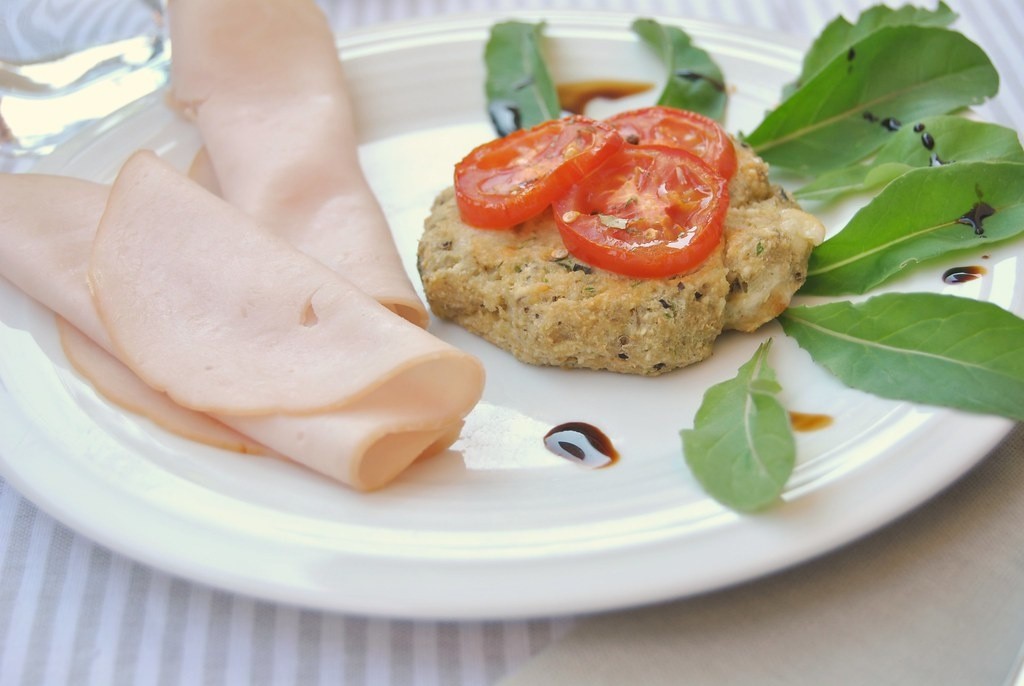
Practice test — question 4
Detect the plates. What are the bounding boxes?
[0,14,1024,625]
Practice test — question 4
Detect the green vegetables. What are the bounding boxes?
[476,1,1024,511]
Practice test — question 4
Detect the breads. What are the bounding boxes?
[415,137,821,375]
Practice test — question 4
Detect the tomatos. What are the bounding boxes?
[451,103,738,278]
